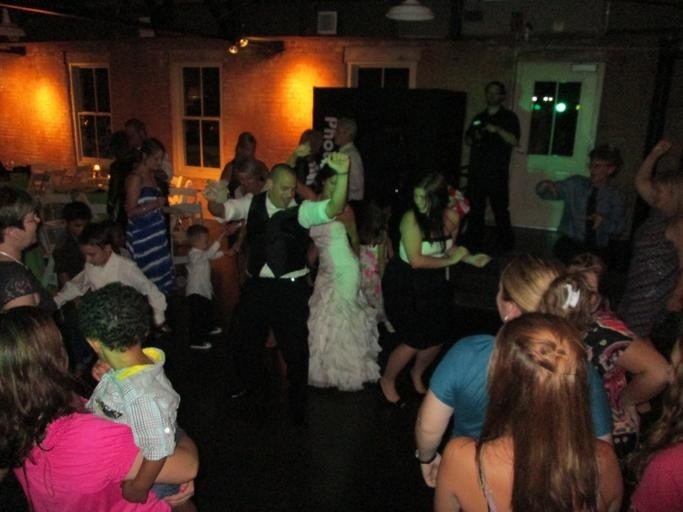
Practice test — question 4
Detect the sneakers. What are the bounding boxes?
[190,341,212,350]
[207,326,222,335]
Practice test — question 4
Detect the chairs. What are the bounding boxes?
[29,163,204,264]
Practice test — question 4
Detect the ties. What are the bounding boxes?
[585,188,597,253]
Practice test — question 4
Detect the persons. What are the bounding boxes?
[208,116,494,428]
[463,83,521,252]
[415,141,681,512]
[184,224,236,350]
[2,118,199,512]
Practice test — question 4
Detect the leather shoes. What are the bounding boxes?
[374,379,409,410]
